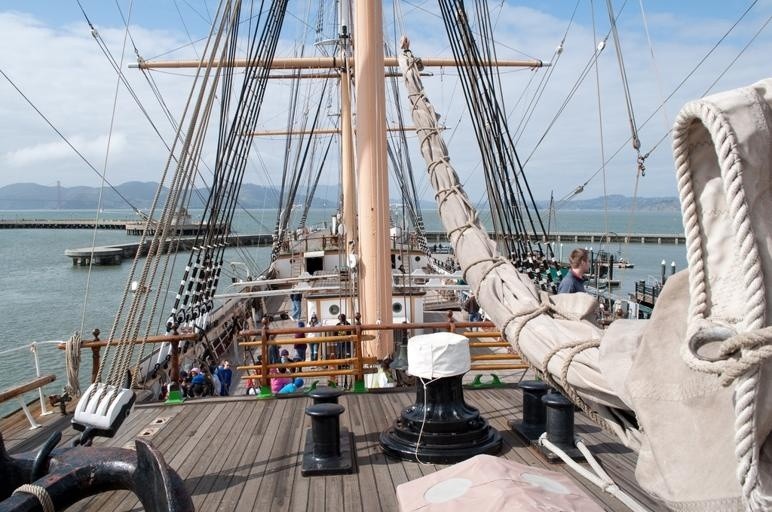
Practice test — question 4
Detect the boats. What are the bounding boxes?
[590,256,634,269]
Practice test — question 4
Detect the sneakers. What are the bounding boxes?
[310,366,317,369]
[289,315,303,321]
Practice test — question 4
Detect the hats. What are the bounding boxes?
[191,368,200,374]
[281,349,289,355]
[311,312,317,322]
[298,321,304,327]
[295,378,303,387]
[293,355,302,360]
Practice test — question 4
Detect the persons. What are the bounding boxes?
[159,312,351,403]
[555,247,591,291]
[289,288,302,322]
[463,293,481,332]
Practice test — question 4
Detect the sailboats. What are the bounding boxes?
[0,0,772,512]
